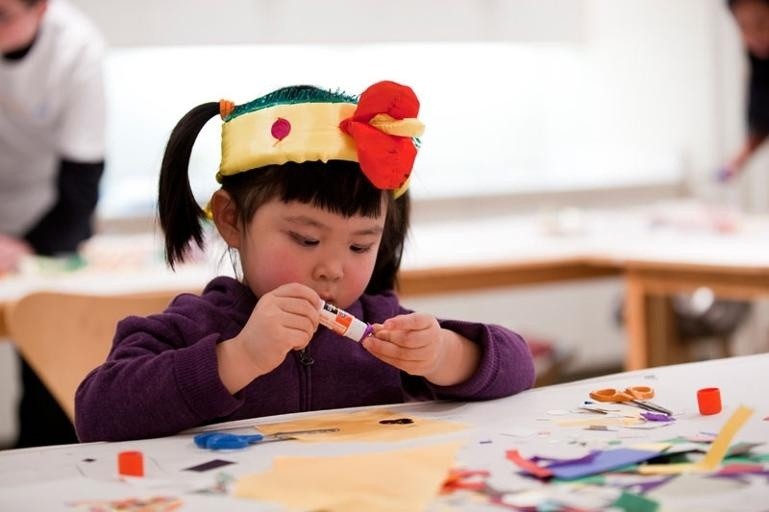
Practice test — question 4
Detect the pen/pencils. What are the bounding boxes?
[308,299,372,345]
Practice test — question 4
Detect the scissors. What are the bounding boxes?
[194,429,338,449]
[589,386,673,416]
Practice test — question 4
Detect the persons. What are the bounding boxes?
[0,0,115,451]
[718,1,768,211]
[72,79,537,444]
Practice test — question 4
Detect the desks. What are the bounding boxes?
[0,202,769,512]
